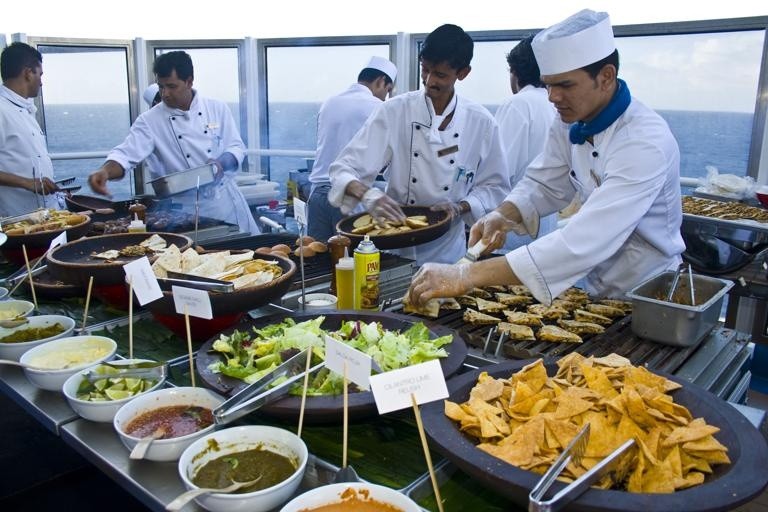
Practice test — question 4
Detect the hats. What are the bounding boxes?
[365,56,397,84]
[143,83,159,107]
[530,9,616,76]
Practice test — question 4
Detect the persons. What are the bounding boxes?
[309,68,392,242]
[493,34,562,253]
[0,43,62,214]
[328,23,512,265]
[408,50,688,307]
[90,51,260,237]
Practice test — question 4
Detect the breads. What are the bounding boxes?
[254,236,329,259]
[402,284,633,344]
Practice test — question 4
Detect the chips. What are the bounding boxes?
[445,352,732,493]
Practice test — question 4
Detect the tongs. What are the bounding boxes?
[85,353,163,384]
[666,259,699,307]
[528,424,634,511]
[4,259,48,289]
[152,269,237,293]
[39,176,83,197]
[210,345,334,424]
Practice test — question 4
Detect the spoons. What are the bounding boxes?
[167,466,262,512]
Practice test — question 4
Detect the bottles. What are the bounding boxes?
[129,198,148,234]
[328,234,380,311]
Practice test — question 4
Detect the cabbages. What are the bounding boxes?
[211,315,453,399]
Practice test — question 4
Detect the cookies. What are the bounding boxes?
[350,212,430,237]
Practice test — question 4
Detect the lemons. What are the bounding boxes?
[78,365,153,402]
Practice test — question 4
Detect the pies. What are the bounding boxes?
[151,244,283,290]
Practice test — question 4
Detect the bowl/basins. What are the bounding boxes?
[1,315,76,362]
[333,207,453,248]
[194,310,477,410]
[1,287,9,299]
[145,250,294,338]
[177,424,310,511]
[46,228,193,284]
[296,293,337,310]
[113,385,230,464]
[64,357,167,424]
[5,211,91,247]
[280,481,424,512]
[19,335,118,392]
[423,355,764,512]
[0,300,36,322]
